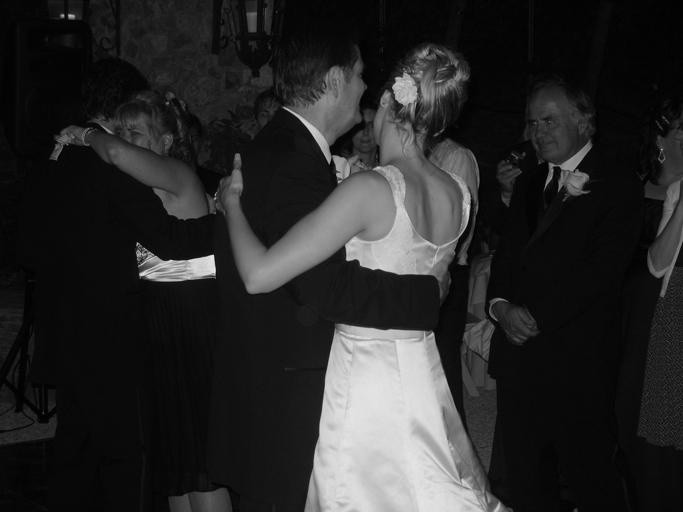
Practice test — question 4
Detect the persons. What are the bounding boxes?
[0,1,683,512]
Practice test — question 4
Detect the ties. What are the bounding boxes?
[544,167,561,207]
